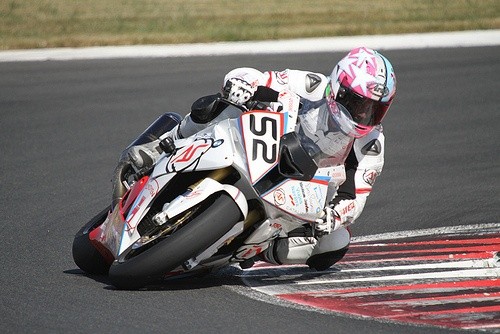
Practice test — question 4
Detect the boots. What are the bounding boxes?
[128,120,182,171]
[239,238,283,269]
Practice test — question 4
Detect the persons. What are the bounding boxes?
[125,46,397,270]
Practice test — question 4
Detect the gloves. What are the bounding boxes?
[312,205,341,234]
[224,77,256,105]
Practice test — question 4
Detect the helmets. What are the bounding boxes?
[323,47,396,138]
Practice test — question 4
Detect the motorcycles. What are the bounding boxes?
[72,86,351,285]
